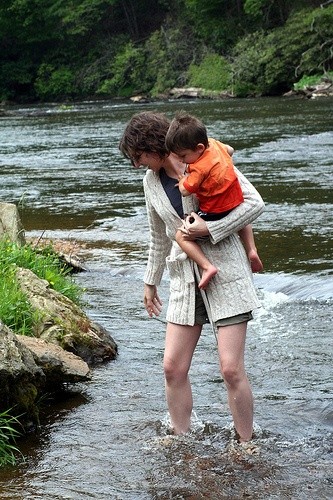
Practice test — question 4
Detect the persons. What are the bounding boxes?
[164,109,263,289]
[119,110,266,445]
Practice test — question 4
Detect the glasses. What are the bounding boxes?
[130,151,143,161]
